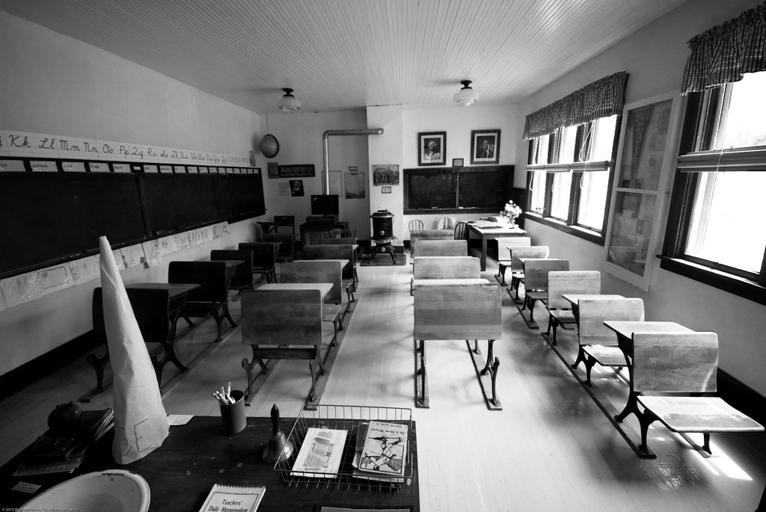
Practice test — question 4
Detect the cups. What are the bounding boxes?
[220,390,246,434]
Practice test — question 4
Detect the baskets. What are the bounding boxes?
[273,405,412,496]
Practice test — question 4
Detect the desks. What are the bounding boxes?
[0,411,420,512]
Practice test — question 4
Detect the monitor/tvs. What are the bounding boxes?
[311,195,339,218]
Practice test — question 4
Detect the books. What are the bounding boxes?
[352,420,409,483]
[289,427,348,479]
[198,483,267,512]
[22,405,114,461]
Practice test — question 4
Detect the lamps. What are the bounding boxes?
[278,88,303,113]
[453,80,480,107]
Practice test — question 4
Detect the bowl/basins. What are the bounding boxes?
[15,469,153,512]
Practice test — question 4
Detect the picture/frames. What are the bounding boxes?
[418,131,446,166]
[453,158,464,167]
[470,129,500,164]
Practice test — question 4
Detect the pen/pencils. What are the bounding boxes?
[212,380,235,405]
